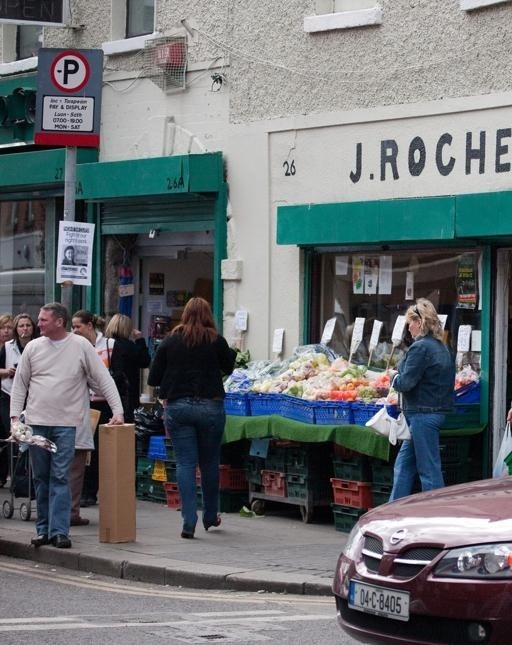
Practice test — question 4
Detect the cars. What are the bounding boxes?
[332,475,512,640]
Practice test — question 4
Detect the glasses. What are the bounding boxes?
[413,305,421,319]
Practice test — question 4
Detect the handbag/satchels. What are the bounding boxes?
[365,408,411,445]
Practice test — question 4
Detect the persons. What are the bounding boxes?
[146,297,236,539]
[0,300,152,549]
[506,402,512,426]
[61,246,77,265]
[386,297,455,502]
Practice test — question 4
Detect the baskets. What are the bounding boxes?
[453,381,480,409]
[244,440,322,498]
[135,437,232,512]
[329,438,472,535]
[223,392,397,425]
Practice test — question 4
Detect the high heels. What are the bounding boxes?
[181,517,221,539]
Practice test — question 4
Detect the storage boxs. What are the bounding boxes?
[98,422,137,545]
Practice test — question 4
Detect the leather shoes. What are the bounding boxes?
[31,517,89,548]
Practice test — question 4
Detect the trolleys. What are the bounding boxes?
[3,410,36,521]
